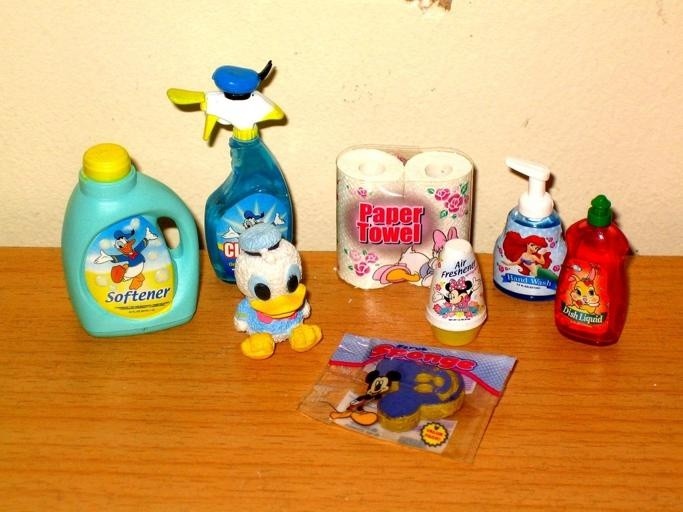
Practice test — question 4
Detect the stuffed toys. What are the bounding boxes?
[231,223,324,361]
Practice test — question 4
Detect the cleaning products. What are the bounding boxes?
[555,196,634,344]
[165,61,293,283]
[61,145,200,338]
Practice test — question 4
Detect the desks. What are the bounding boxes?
[0,248,683,512]
